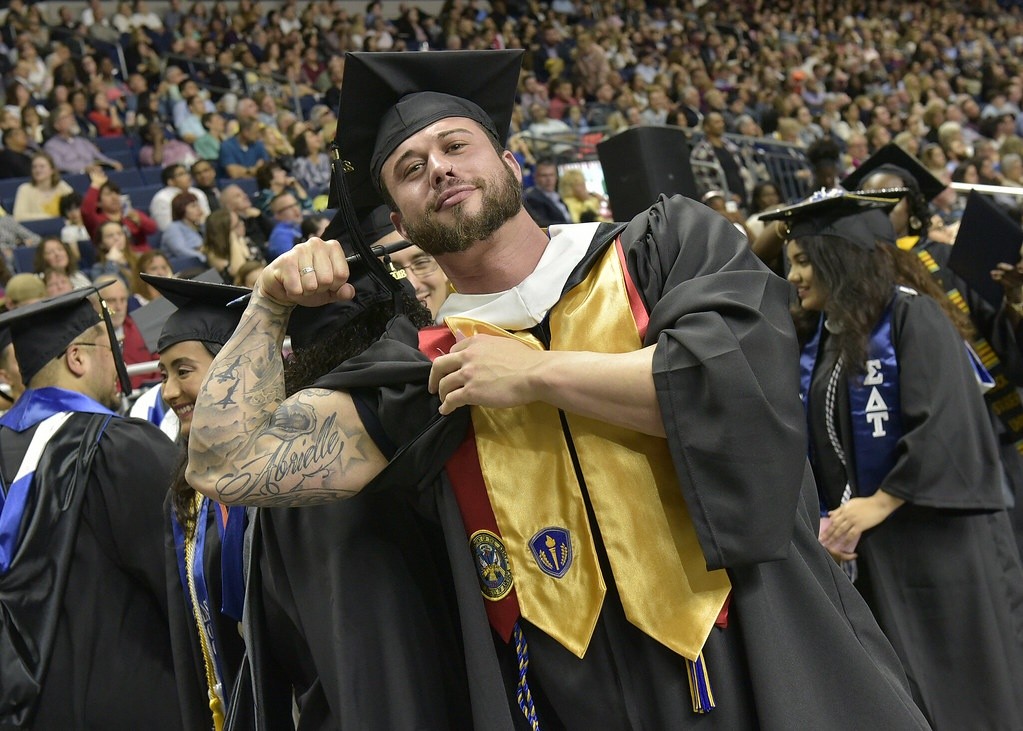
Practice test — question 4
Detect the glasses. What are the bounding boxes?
[279,202,301,216]
[387,256,438,275]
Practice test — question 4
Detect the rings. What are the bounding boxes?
[300,266,315,277]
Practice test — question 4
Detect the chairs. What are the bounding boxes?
[0,133,255,277]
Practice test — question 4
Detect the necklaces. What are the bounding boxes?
[823,333,846,464]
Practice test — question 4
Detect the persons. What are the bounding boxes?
[182,49,931,731]
[757,184,1023,730]
[0,282,186,730]
[1,1,1022,730]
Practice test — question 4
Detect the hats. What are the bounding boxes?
[839,142,947,204]
[758,194,899,248]
[0,273,48,307]
[226,243,415,352]
[319,208,396,255]
[327,49,524,295]
[139,272,252,354]
[0,280,133,396]
[946,187,1023,308]
